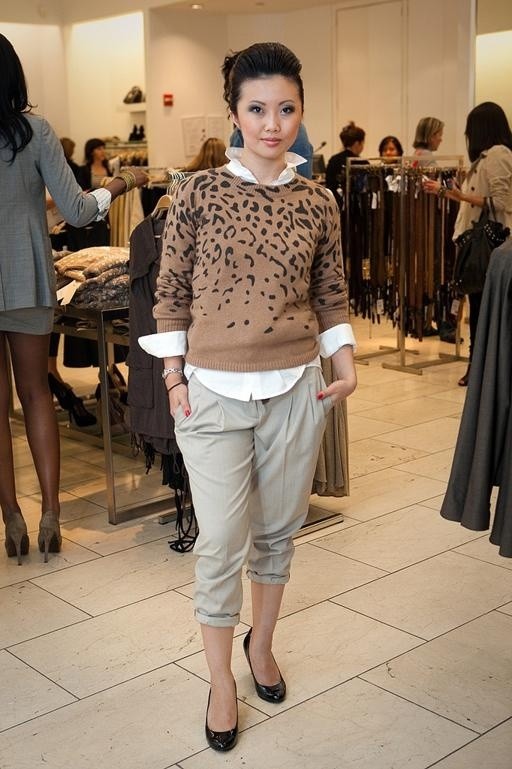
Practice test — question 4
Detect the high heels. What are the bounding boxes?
[5,511,30,566]
[37,510,63,563]
[48,371,98,428]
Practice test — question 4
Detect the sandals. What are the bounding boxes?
[458,373,469,386]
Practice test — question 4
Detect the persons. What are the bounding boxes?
[0,30,150,569]
[183,132,229,175]
[376,135,405,281]
[135,40,363,755]
[57,137,87,193]
[79,138,114,191]
[422,99,512,388]
[323,118,372,310]
[411,116,447,179]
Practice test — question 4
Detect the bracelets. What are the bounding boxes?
[437,185,448,199]
[166,380,185,394]
[160,364,185,379]
[112,171,137,194]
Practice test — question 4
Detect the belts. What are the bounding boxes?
[348,164,460,343]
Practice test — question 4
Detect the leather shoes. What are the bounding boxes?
[242,627,287,704]
[204,677,240,750]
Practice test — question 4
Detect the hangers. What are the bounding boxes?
[150,171,179,238]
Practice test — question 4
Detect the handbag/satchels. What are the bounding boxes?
[453,218,510,285]
[123,86,142,103]
